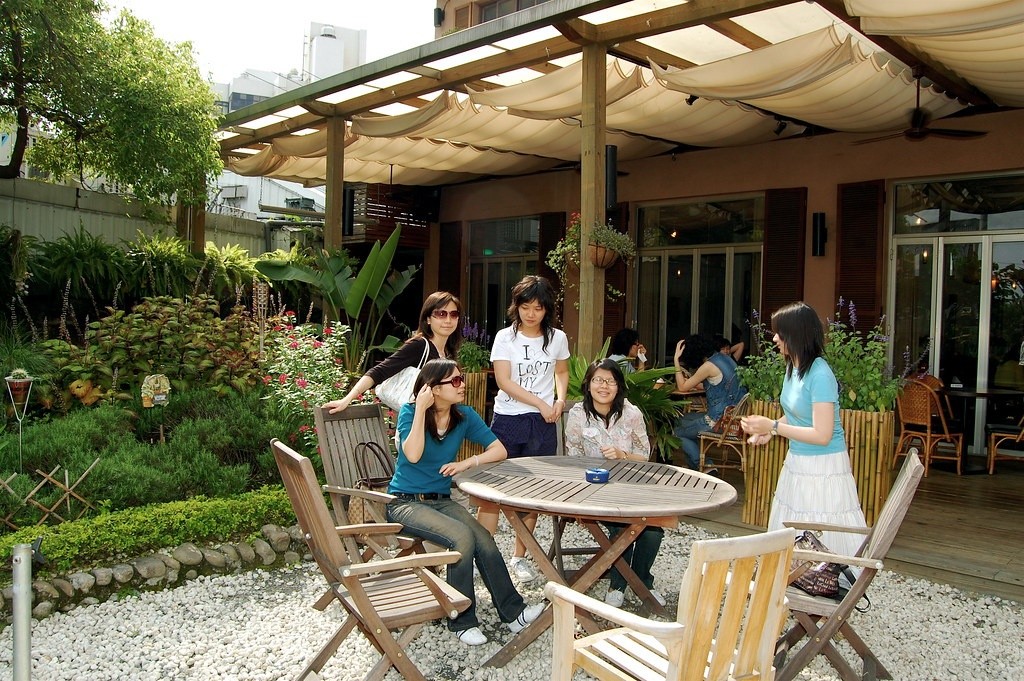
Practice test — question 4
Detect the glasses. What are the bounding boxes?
[590,376,618,386]
[437,374,465,387]
[634,340,641,347]
[432,309,460,319]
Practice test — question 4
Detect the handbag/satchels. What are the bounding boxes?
[713,405,742,437]
[374,336,429,412]
[346,442,395,524]
[787,530,872,613]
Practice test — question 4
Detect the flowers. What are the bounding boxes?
[455,316,492,371]
[544,211,581,270]
[732,296,910,411]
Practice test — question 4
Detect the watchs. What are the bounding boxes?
[771,420,779,436]
[622,451,628,460]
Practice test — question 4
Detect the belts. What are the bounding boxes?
[391,492,451,502]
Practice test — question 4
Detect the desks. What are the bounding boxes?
[654,382,707,413]
[931,385,1024,475]
[454,456,738,669]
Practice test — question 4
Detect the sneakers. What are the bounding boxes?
[648,589,666,607]
[510,557,536,583]
[455,626,487,645]
[506,603,545,634]
[605,588,624,607]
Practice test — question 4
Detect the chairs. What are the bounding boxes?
[270,437,472,681]
[697,392,751,487]
[891,374,963,475]
[749,447,925,681]
[984,416,1024,475]
[543,526,794,680]
[553,399,579,456]
[314,402,446,610]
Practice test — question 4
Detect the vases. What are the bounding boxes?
[564,250,580,276]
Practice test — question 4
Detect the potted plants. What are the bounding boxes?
[585,211,637,268]
[5,368,33,405]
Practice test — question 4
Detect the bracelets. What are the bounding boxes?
[557,400,565,408]
[675,369,682,373]
[473,455,479,466]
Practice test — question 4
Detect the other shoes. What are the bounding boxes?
[702,467,718,476]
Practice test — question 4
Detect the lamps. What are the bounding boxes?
[811,211,828,257]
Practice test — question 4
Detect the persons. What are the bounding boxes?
[472,275,571,581]
[323,292,469,574]
[565,358,667,608]
[741,301,870,642]
[608,327,647,373]
[387,360,546,644]
[673,334,747,475]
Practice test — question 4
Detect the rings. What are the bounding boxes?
[549,419,551,421]
